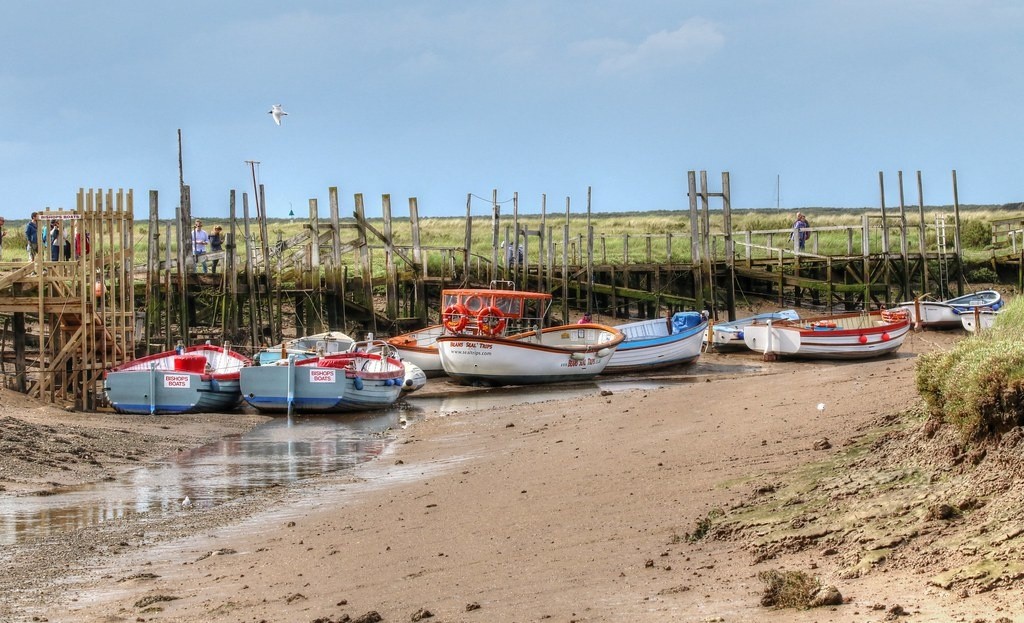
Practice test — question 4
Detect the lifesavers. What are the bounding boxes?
[813,322,836,327]
[881,308,908,323]
[443,297,504,334]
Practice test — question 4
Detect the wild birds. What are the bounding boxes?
[268,104,288,126]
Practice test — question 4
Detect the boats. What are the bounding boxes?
[701,308,803,353]
[901,290,1004,330]
[238,343,403,414]
[253,332,359,372]
[441,289,627,385]
[103,341,253,414]
[362,348,430,395]
[960,305,1007,334]
[599,312,716,374]
[373,315,487,376]
[745,306,914,360]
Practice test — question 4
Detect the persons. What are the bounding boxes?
[0,216,6,277]
[190,218,208,273]
[209,225,224,273]
[24,212,90,274]
[500,241,524,265]
[787,211,811,252]
